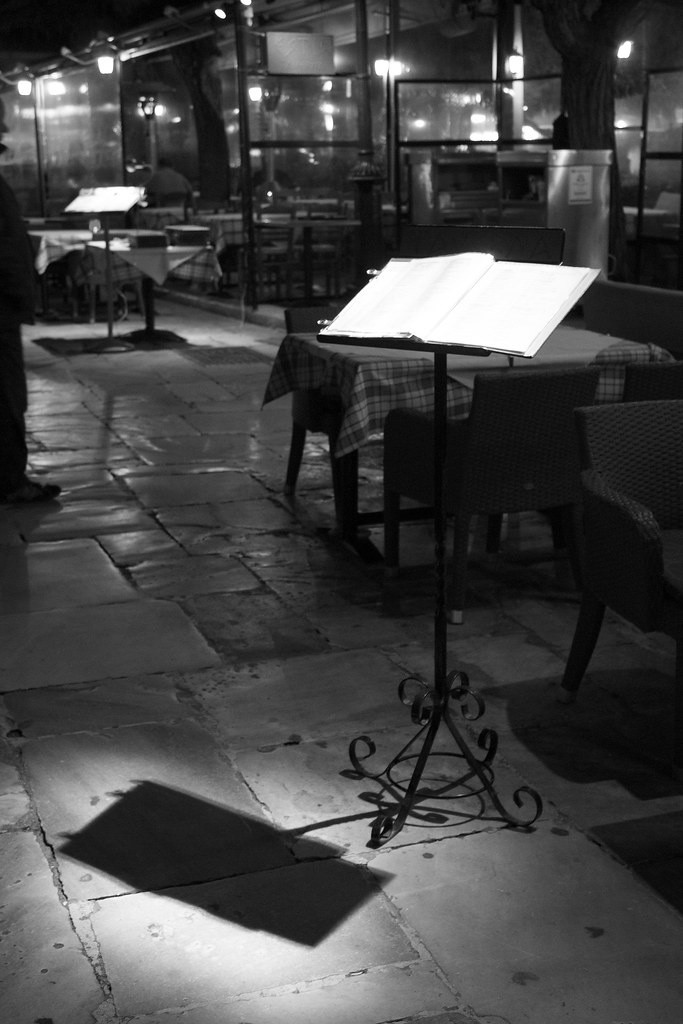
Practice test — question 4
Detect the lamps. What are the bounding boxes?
[88,30,121,75]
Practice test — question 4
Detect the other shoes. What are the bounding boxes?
[0,481,61,504]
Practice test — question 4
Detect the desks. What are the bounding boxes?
[23,196,677,575]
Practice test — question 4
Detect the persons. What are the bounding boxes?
[0,96,60,502]
[145,157,192,194]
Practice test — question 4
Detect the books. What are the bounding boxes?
[318,251,602,359]
[63,183,146,212]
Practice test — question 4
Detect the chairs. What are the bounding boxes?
[283,305,337,497]
[623,362,683,403]
[385,365,600,626]
[557,398,683,770]
